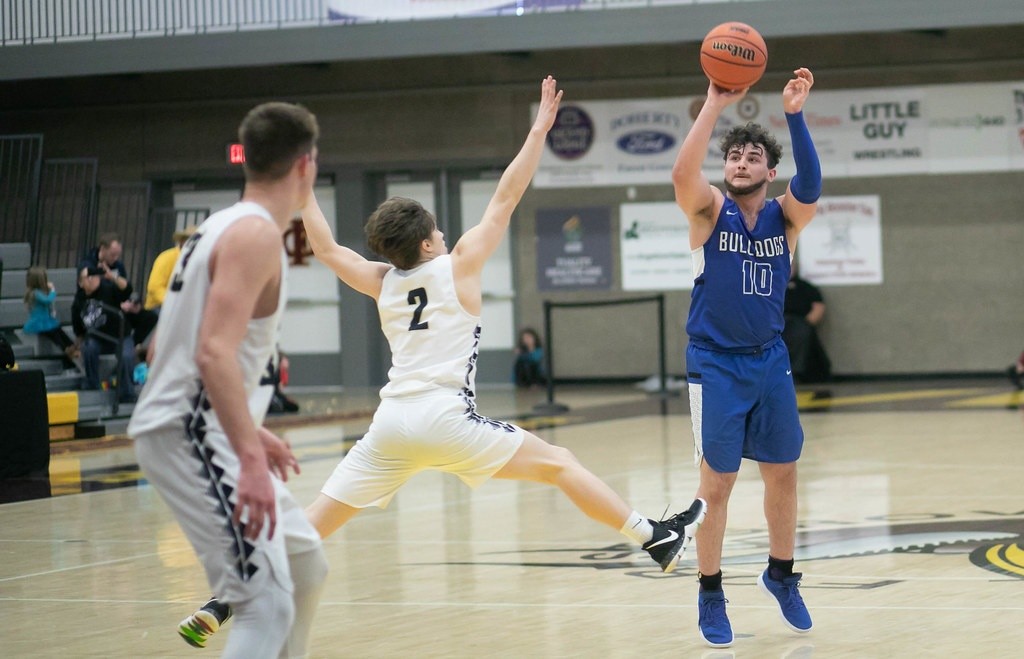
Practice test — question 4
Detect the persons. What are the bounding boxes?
[71,226,200,403]
[176,74,708,649]
[672,68,821,648]
[512,328,549,391]
[260,344,300,415]
[126,103,328,659]
[23,267,81,374]
[782,261,833,400]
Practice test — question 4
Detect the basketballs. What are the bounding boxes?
[699,20,770,92]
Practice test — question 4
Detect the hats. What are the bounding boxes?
[172,224,198,243]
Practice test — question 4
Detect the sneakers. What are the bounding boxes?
[641,497,708,574]
[176,596,233,649]
[757,564,814,634]
[696,585,735,649]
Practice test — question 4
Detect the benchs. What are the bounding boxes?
[0,243,151,421]
